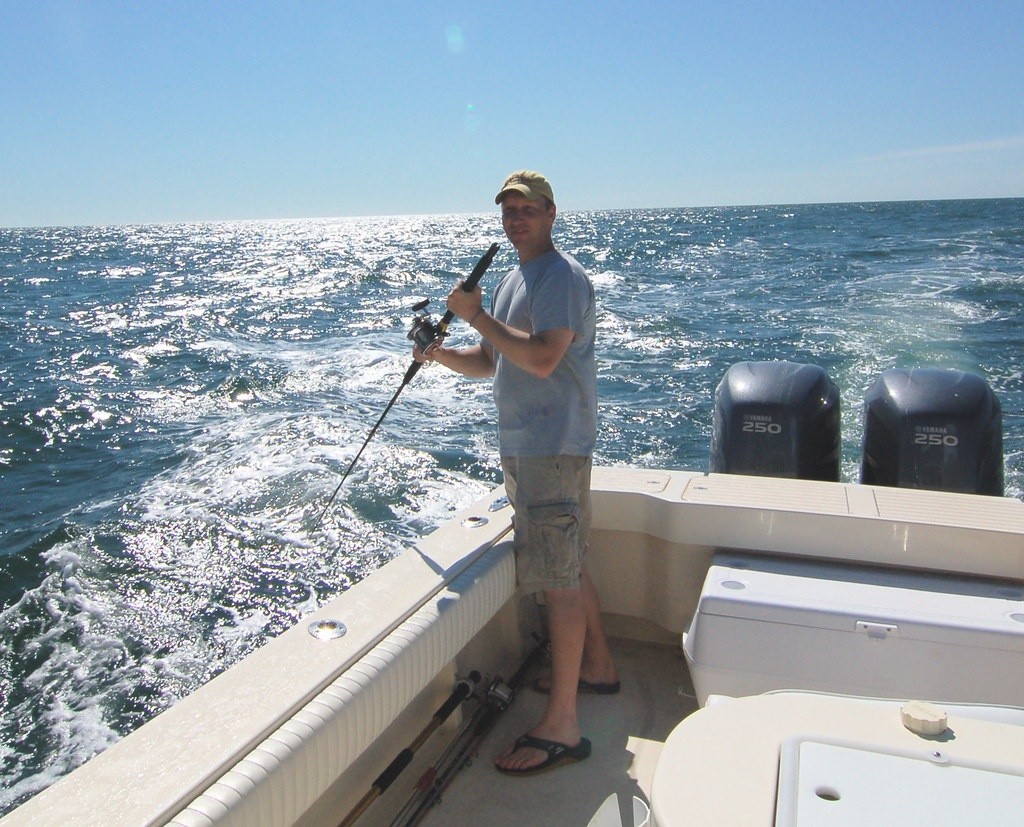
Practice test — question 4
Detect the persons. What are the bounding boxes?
[411,167,623,778]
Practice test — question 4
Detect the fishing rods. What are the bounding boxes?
[308,241,502,537]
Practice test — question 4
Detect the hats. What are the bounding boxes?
[495,170,555,205]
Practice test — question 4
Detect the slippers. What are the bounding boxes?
[533,672,621,696]
[495,734,591,776]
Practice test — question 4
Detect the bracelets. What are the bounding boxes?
[469,308,485,327]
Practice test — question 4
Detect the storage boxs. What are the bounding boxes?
[681,549,1024,709]
